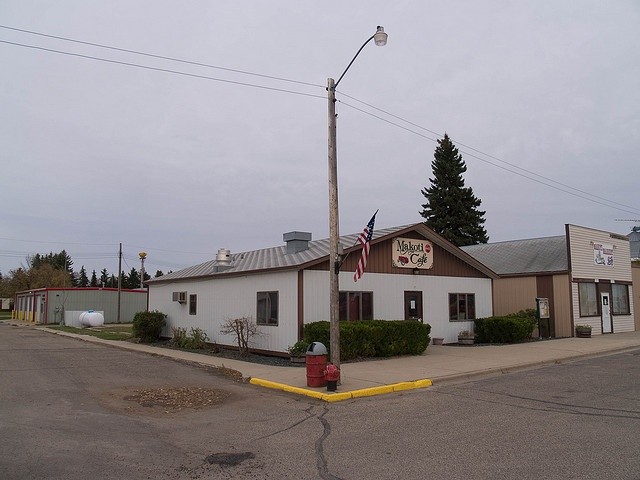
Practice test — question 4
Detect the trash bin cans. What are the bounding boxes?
[306,342,328,386]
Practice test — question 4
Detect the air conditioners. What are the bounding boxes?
[173,290,187,303]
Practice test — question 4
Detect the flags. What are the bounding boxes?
[353,213,378,283]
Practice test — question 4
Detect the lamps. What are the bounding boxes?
[412,266,420,275]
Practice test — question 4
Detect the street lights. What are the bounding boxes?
[326,26,390,384]
[139,252,148,291]
